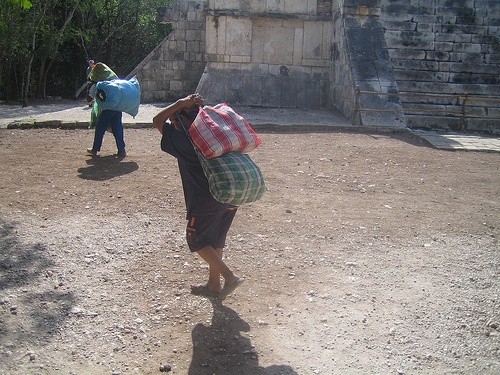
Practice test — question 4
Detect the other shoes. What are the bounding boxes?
[87,148,97,155]
[113,153,126,157]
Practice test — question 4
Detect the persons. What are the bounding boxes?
[153,93,245,300]
[87,82,127,159]
[86,60,96,107]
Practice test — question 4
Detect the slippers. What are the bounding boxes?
[191,282,219,296]
[219,277,245,299]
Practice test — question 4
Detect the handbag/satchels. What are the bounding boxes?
[175,111,265,205]
[187,103,262,159]
